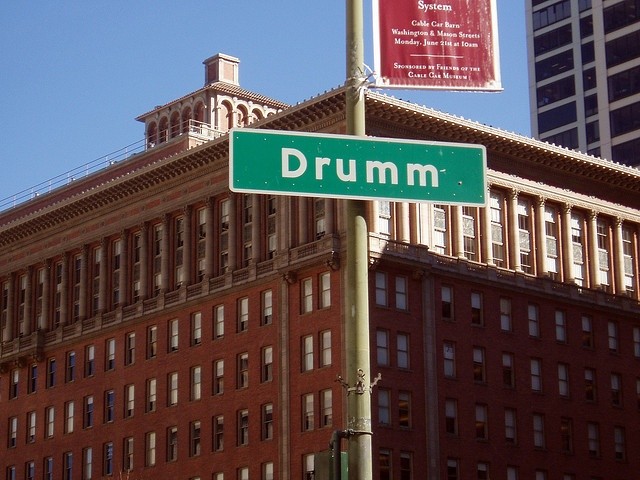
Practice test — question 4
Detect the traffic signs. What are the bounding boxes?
[229,127,489,210]
